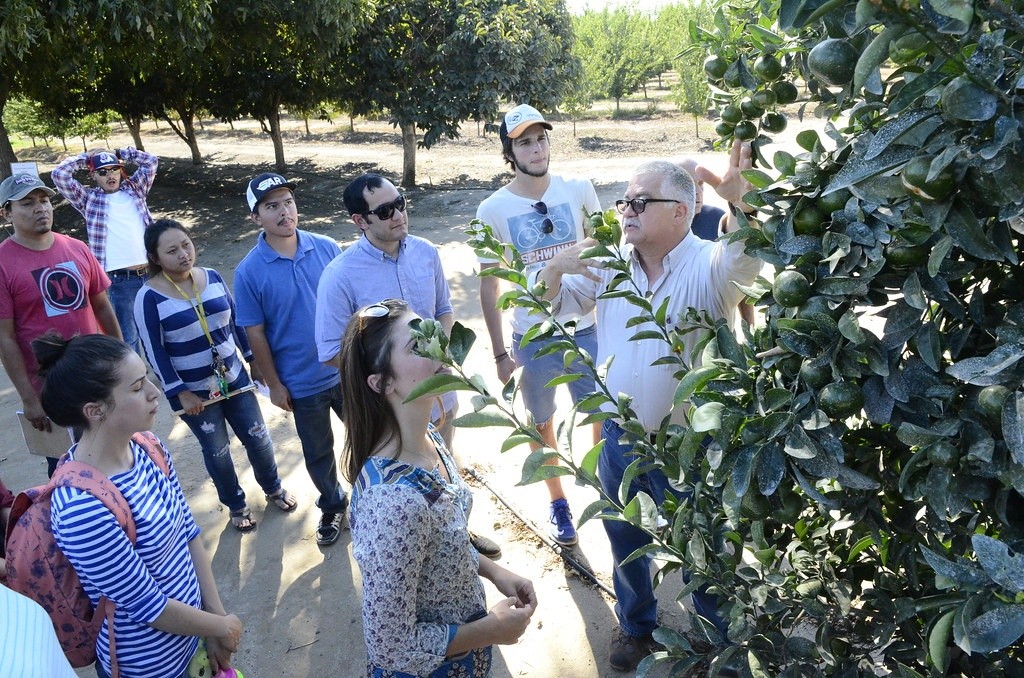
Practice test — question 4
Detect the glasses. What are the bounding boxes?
[362,194,407,220]
[531,201,554,234]
[93,167,120,176]
[358,299,399,355]
[616,198,680,214]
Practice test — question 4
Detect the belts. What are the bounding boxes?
[107,266,150,277]
[612,421,671,446]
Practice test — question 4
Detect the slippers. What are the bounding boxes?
[229,508,256,530]
[264,488,297,511]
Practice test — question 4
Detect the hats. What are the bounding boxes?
[499,104,553,141]
[247,172,297,211]
[0,173,56,207]
[90,153,123,170]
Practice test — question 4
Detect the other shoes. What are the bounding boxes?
[609,628,649,670]
[470,534,501,558]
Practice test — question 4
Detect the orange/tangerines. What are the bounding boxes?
[700,38,1010,677]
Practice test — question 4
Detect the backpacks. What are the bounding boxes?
[3,432,168,668]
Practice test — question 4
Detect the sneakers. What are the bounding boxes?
[549,499,577,544]
[314,511,345,545]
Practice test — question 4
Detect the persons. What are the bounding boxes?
[475,103,606,544]
[340,297,537,678]
[314,175,502,557]
[529,141,767,670]
[674,159,754,334]
[29,330,241,678]
[132,218,298,532]
[50,146,158,358]
[0,173,125,479]
[234,172,350,545]
[0,480,16,588]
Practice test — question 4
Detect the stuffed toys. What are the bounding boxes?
[188,636,244,678]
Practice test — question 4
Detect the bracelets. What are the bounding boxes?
[727,202,757,221]
[494,353,508,364]
